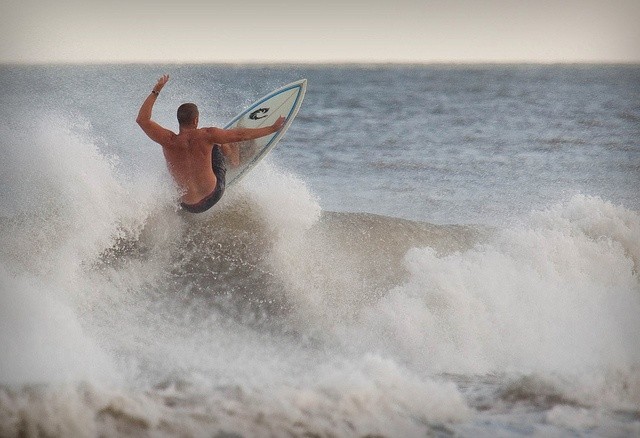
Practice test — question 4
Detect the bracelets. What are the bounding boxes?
[151,91,159,96]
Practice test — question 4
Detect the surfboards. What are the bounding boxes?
[217,78,308,188]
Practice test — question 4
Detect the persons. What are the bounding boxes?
[136,74,285,213]
